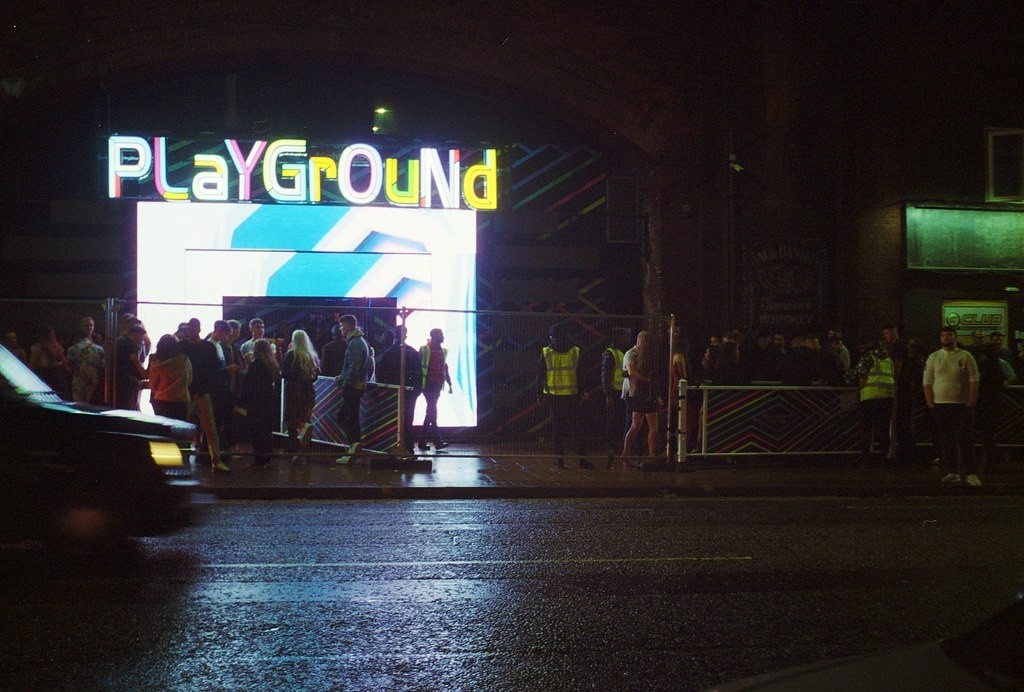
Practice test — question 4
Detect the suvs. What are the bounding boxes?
[1,343,206,562]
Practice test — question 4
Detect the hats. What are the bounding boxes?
[549,323,566,337]
[611,328,631,349]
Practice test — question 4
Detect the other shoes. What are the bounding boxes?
[940,472,961,482]
[436,442,448,450]
[245,462,272,470]
[345,442,363,466]
[223,454,242,460]
[212,463,230,471]
[336,456,362,465]
[553,459,563,467]
[623,463,640,470]
[578,460,592,467]
[418,444,430,449]
[607,463,615,470]
[291,456,308,466]
[966,474,983,486]
[299,422,314,450]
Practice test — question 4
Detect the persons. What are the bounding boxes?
[0,287,1023,488]
